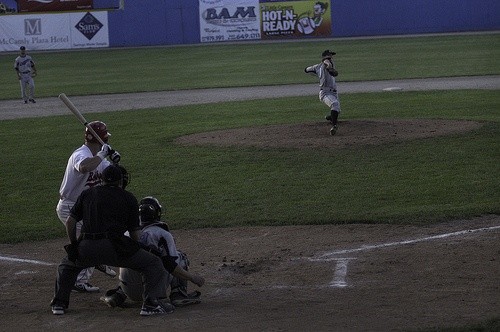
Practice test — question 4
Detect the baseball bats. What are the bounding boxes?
[59,93,115,155]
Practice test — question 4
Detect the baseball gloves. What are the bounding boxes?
[326,65,339,77]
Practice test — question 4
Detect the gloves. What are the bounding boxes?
[96,144,112,161]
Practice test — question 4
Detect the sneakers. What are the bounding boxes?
[50,298,68,315]
[140,299,175,316]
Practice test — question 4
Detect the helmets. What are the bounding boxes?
[321,50,336,58]
[137,196,162,222]
[85,121,112,143]
[20,46,25,50]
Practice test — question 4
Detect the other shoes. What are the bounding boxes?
[329,125,338,136]
[29,98,36,103]
[98,289,127,308]
[71,282,100,293]
[24,100,27,104]
[95,264,117,277]
[325,115,332,120]
[170,286,202,307]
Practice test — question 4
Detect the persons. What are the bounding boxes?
[49,121,204,316]
[305,49,342,135]
[13,46,38,103]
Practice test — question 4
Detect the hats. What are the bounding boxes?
[100,164,123,184]
